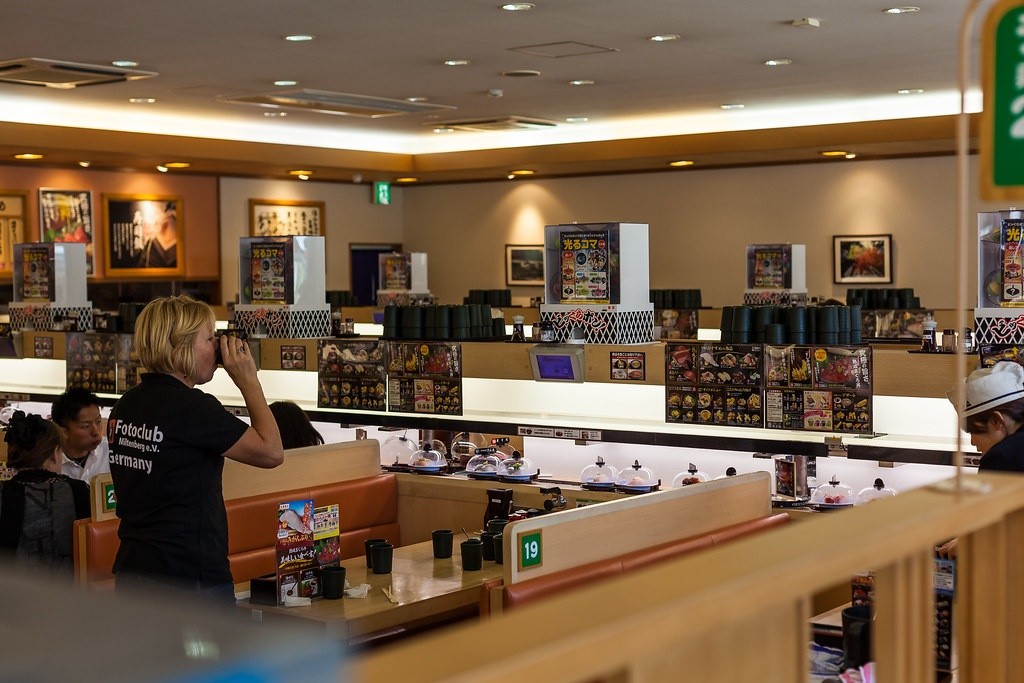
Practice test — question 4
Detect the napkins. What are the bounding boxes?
[284,596,311,606]
[344,584,372,598]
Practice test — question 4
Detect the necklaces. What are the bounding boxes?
[64,449,88,466]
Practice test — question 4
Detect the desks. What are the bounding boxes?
[234,529,503,651]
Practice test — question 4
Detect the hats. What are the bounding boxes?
[946,360,1023,418]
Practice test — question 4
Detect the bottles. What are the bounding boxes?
[532,321,554,341]
[512,315,525,341]
[530,297,542,307]
[410,295,437,305]
[921,312,972,352]
[810,294,826,304]
[53,309,77,329]
[332,311,354,334]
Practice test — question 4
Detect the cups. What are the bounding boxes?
[463,289,512,306]
[326,290,366,307]
[846,288,921,308]
[432,529,454,558]
[650,289,701,307]
[214,346,231,365]
[720,305,862,344]
[460,510,549,572]
[365,538,392,574]
[92,301,150,334]
[383,305,505,336]
[320,566,347,599]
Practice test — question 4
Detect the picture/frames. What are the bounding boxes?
[101,192,186,277]
[833,234,893,284]
[0,188,40,280]
[505,244,545,287]
[39,187,96,278]
[249,198,325,236]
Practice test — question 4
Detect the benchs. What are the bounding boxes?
[74,474,401,598]
[479,511,791,616]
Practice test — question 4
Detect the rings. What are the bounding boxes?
[236,344,245,352]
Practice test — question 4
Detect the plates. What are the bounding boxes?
[581,483,660,494]
[381,459,539,483]
[771,496,851,510]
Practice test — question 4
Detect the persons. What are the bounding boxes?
[945,362,1024,474]
[107,295,284,669]
[0,411,90,596]
[268,400,324,450]
[51,387,111,487]
